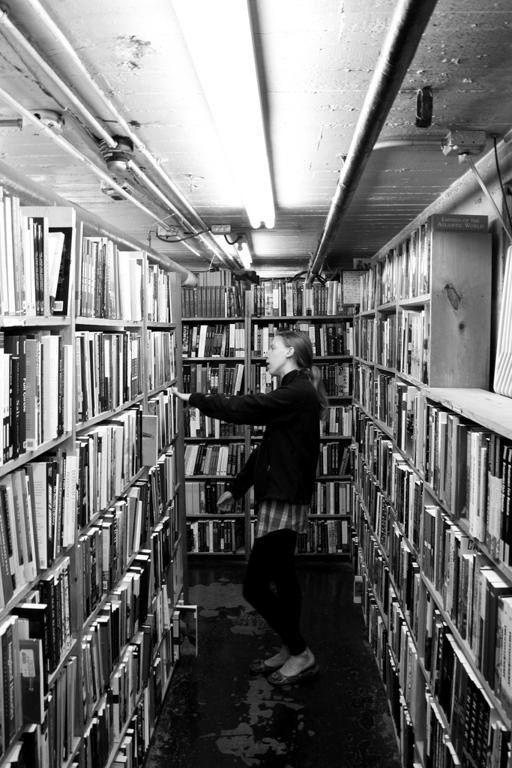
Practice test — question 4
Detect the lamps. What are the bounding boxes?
[167,0,276,231]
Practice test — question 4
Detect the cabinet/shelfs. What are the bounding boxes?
[0,192,185,768]
[356,212,512,768]
[182,279,356,560]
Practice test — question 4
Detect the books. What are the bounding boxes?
[173,221,434,358]
[9,185,167,758]
[333,356,512,754]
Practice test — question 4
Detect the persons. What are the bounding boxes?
[159,331,334,686]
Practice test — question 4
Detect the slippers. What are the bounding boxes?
[249,659,280,673]
[267,666,319,687]
